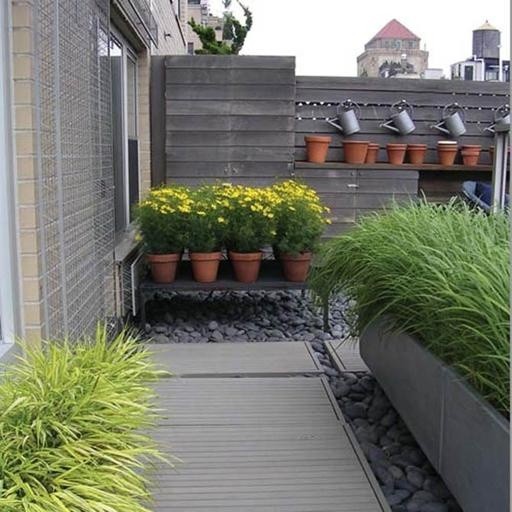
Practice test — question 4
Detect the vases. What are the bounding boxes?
[282,252,311,282]
[229,251,263,282]
[145,254,180,284]
[191,252,222,283]
[305,136,510,167]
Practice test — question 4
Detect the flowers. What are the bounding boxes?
[131,176,332,259]
